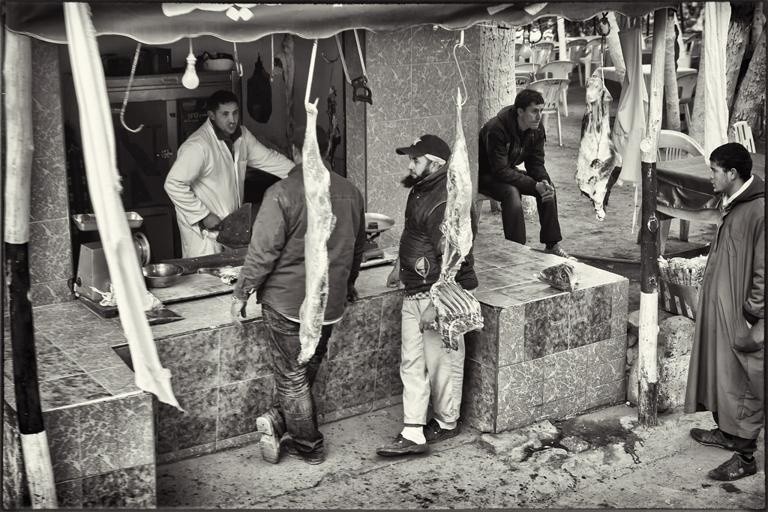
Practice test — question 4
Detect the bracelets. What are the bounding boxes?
[142,262,185,288]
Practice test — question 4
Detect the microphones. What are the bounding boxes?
[472,187,501,232]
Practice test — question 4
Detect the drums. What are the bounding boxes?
[215,202,253,249]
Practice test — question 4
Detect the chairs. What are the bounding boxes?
[544,244,567,256]
[690,428,758,452]
[708,453,757,480]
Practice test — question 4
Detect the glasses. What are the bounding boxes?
[182,38,200,89]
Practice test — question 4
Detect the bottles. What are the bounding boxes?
[289,125,330,154]
[395,135,451,162]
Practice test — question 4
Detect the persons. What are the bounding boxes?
[230,122,366,465]
[164,89,296,260]
[682,143,766,482]
[479,87,577,263]
[376,133,479,459]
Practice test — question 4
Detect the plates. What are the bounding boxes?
[107,69,242,264]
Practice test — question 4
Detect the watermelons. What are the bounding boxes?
[361,213,395,263]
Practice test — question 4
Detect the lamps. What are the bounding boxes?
[677,71,698,128]
[535,60,575,78]
[644,34,654,49]
[566,39,589,88]
[530,42,554,62]
[515,63,539,79]
[636,129,707,255]
[523,78,570,146]
[577,38,605,87]
[732,121,756,153]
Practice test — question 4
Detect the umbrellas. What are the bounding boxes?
[378,432,430,458]
[256,410,286,465]
[427,428,463,441]
[282,438,327,463]
